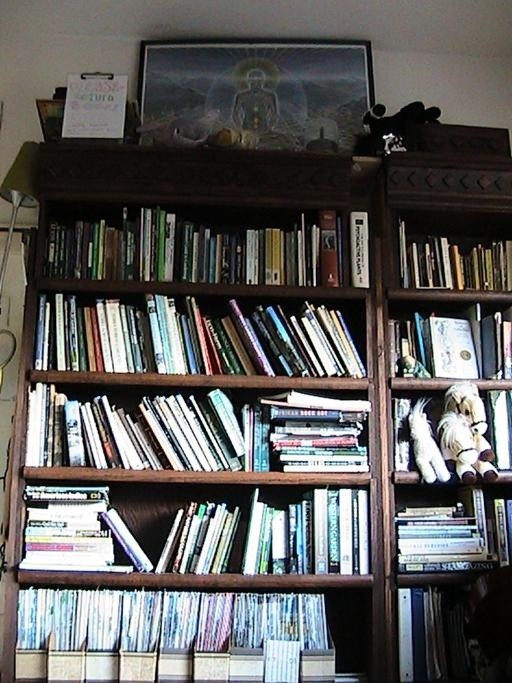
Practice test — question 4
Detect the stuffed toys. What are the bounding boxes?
[408,385,500,487]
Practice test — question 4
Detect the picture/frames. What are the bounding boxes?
[135,36,376,156]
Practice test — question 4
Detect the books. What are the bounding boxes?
[398,588,510,683]
[388,303,512,380]
[48,205,370,288]
[394,487,511,572]
[16,590,364,683]
[25,382,371,472]
[397,219,512,292]
[36,294,366,378]
[392,390,511,471]
[19,486,369,576]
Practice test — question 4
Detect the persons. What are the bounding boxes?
[233,68,279,139]
